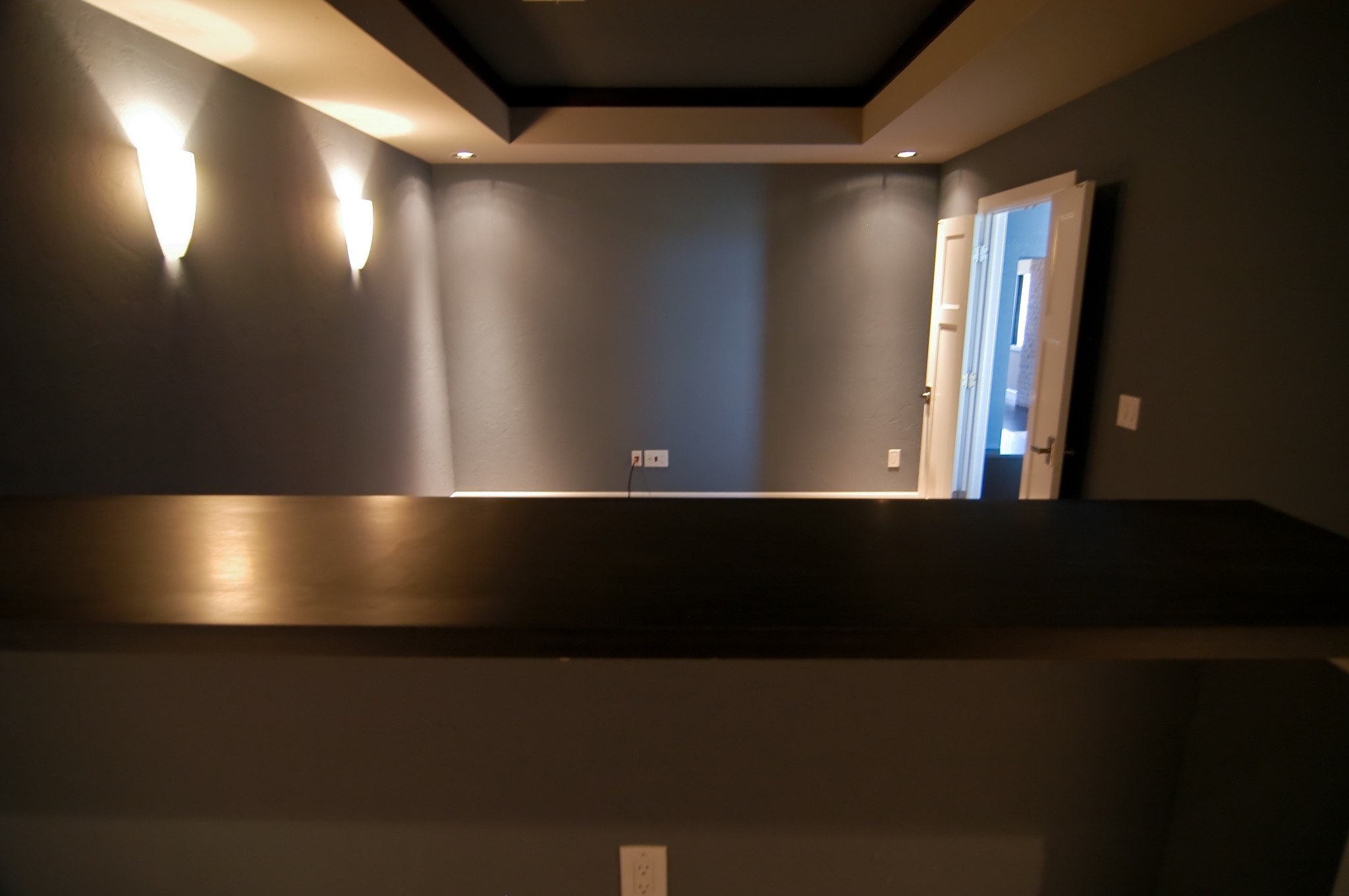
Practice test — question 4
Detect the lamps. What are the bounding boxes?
[341,200,374,271]
[136,144,196,259]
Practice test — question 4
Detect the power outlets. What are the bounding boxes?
[619,845,668,896]
[631,450,643,467]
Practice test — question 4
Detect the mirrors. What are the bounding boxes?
[0,1,1349,632]
